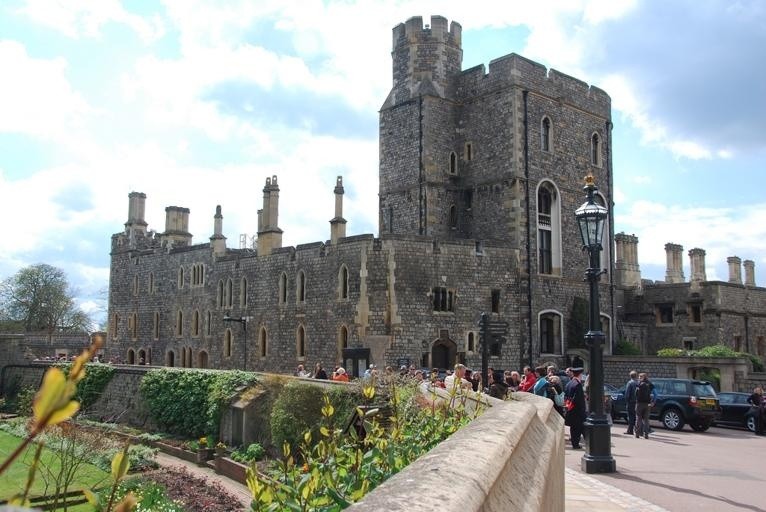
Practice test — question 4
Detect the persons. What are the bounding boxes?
[35,353,146,365]
[747,387,763,436]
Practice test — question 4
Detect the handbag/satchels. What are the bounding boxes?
[554,391,566,407]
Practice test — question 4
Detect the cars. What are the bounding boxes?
[710,392,756,432]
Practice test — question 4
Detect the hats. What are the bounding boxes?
[569,367,584,374]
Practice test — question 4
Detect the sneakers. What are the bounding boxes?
[573,442,586,451]
[623,430,650,439]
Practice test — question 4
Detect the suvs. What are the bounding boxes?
[604,377,721,432]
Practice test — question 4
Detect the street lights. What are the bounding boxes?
[222,317,246,371]
[573,173,618,474]
